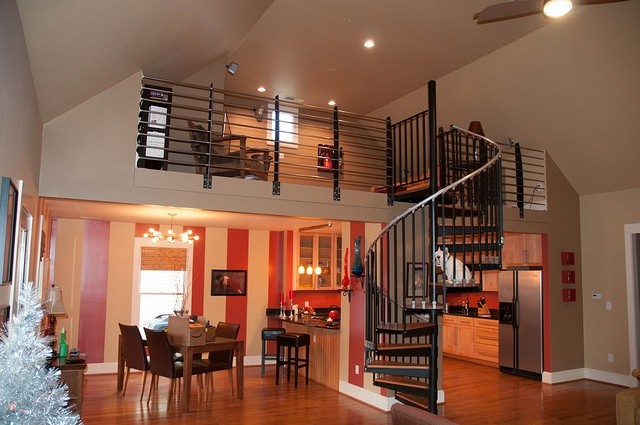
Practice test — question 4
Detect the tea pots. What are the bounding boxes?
[327,304,340,320]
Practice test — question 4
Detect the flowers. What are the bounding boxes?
[301,306,316,315]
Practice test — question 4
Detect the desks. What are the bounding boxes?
[116,333,244,401]
[45,353,88,420]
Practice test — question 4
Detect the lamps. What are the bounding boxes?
[541,0,574,18]
[40,285,68,359]
[143,211,199,245]
[298,230,305,275]
[307,228,313,275]
[315,228,322,275]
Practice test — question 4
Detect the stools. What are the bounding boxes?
[276,332,310,388]
[261,328,285,378]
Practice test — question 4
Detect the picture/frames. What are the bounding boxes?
[210,269,247,296]
[0,303,11,332]
[0,177,19,286]
[407,262,428,298]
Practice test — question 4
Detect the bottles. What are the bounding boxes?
[462,297,465,314]
[457,299,462,314]
[58,326,69,357]
[465,295,470,315]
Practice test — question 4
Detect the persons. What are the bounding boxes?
[214,272,244,295]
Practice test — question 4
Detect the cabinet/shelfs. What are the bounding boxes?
[457,317,475,361]
[293,231,334,291]
[442,315,458,361]
[333,233,341,290]
[475,318,499,368]
[504,232,544,269]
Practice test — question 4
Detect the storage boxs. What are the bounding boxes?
[167,316,206,347]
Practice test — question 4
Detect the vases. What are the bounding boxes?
[303,314,311,322]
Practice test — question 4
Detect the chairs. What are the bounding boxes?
[143,326,208,412]
[187,120,273,181]
[192,321,240,403]
[118,322,156,402]
[173,352,203,394]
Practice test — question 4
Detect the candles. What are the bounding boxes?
[283,301,287,307]
[289,289,294,299]
[280,292,284,306]
